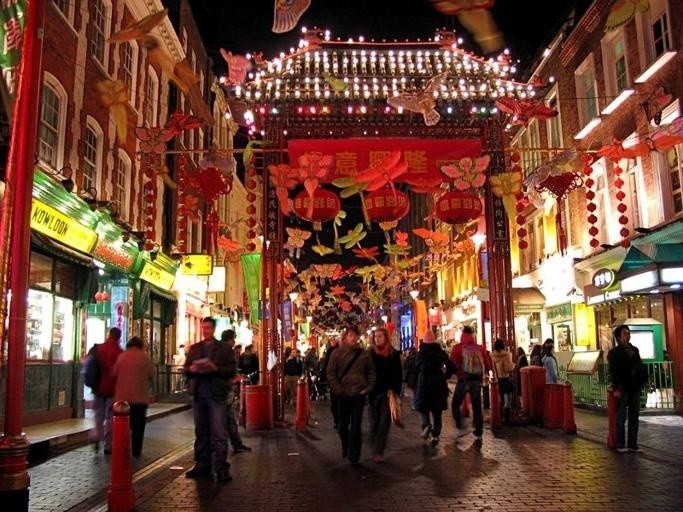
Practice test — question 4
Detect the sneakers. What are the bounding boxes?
[420,425,439,441]
[234,444,251,451]
[185,464,231,481]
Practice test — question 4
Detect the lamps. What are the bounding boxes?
[45,166,160,261]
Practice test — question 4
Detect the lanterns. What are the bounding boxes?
[239,152,259,253]
[291,185,341,232]
[174,154,189,255]
[144,166,158,252]
[435,191,482,234]
[362,187,411,231]
[508,153,528,249]
[579,154,599,248]
[611,154,631,248]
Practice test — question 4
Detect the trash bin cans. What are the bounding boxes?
[245,384,273,432]
[519,365,546,415]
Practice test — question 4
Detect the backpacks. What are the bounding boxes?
[82,344,101,387]
[463,344,485,374]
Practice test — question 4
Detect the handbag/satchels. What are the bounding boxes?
[498,377,514,393]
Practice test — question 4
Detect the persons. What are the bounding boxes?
[605,324,649,453]
[85,325,124,456]
[106,336,154,458]
[409,329,454,442]
[183,316,236,484]
[364,328,403,464]
[234,344,260,385]
[449,325,492,442]
[493,337,517,419]
[404,347,418,409]
[218,329,251,453]
[515,346,529,413]
[323,325,377,464]
[285,346,323,401]
[530,339,561,383]
